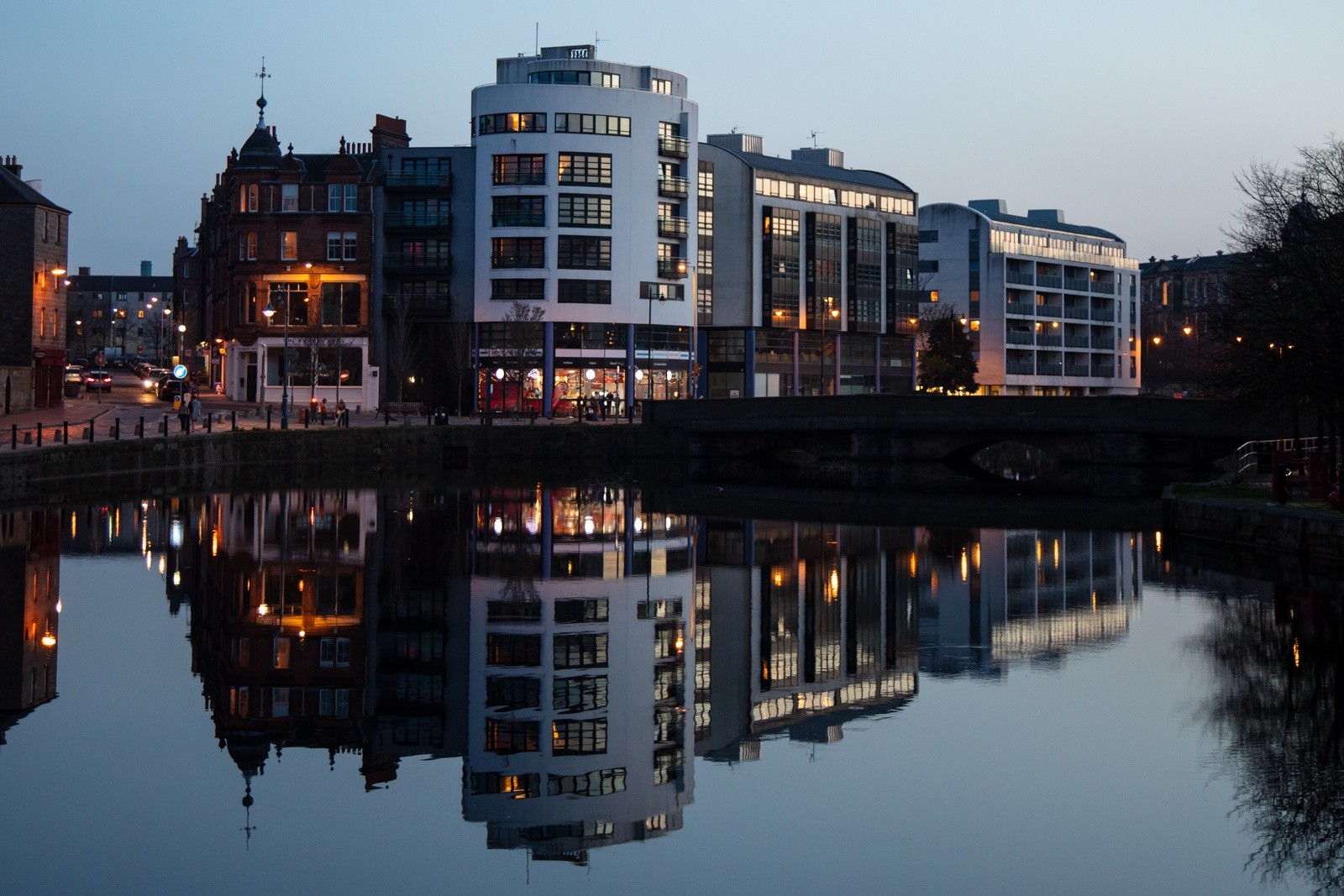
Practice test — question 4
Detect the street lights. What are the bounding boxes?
[951,309,966,387]
[178,325,186,397]
[1183,324,1200,394]
[262,286,289,430]
[820,308,840,396]
[160,309,171,368]
[678,264,697,399]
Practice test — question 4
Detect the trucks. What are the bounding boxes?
[87,347,122,366]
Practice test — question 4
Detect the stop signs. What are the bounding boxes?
[340,370,349,382]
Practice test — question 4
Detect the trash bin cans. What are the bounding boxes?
[434,407,448,424]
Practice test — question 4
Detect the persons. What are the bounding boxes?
[309,397,318,424]
[583,392,620,418]
[320,398,328,425]
[178,395,201,432]
[523,388,539,398]
[337,398,346,427]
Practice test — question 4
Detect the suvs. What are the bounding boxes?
[85,370,113,393]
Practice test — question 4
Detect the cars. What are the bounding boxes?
[65,359,90,385]
[113,355,189,401]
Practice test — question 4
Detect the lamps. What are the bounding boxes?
[35,268,66,284]
